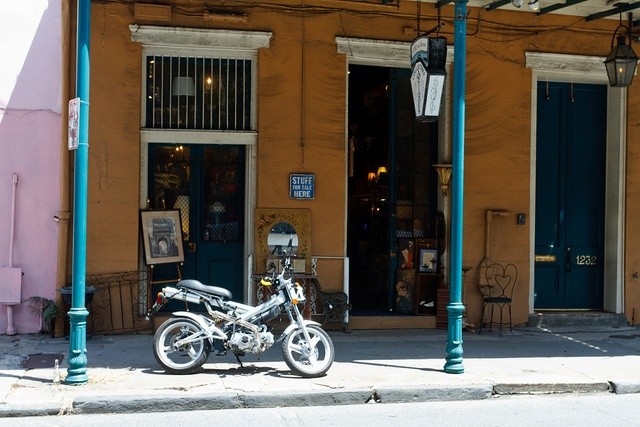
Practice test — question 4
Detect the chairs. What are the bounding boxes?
[480,263,518,335]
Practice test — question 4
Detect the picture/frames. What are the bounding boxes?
[419,249,438,273]
[139,208,185,266]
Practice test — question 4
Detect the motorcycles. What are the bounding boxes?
[145,236,336,378]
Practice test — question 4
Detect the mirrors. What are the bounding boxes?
[254,207,312,276]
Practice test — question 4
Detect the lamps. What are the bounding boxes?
[602,13,639,87]
[172,76,195,97]
[528,0,540,11]
[512,1,524,8]
